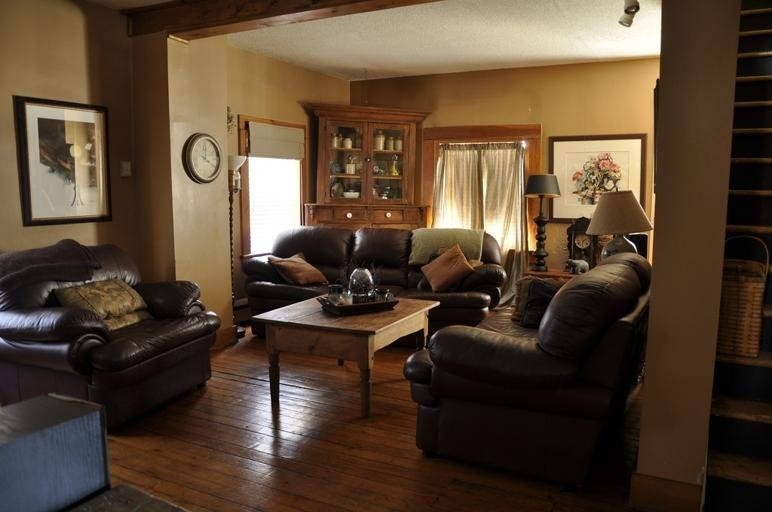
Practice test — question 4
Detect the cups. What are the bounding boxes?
[374,288,393,303]
[328,284,350,306]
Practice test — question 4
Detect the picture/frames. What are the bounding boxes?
[10,95,113,227]
[547,133,648,223]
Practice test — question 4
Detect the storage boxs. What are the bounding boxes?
[717,256,768,357]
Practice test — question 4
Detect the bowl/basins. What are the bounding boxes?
[343,190,361,199]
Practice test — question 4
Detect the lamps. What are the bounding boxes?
[617,1,640,28]
[585,187,652,259]
[227,153,247,338]
[523,175,560,271]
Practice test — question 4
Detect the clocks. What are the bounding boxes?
[181,132,225,185]
[568,218,598,270]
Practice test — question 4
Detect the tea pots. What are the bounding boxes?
[348,257,374,295]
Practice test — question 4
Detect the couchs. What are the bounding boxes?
[240,228,507,338]
[402,252,651,504]
[0,238,223,431]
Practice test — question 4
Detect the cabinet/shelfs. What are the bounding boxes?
[295,100,434,229]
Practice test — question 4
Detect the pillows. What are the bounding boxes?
[54,279,148,320]
[103,311,164,331]
[420,243,475,294]
[267,251,329,286]
[408,228,484,264]
[511,276,565,326]
